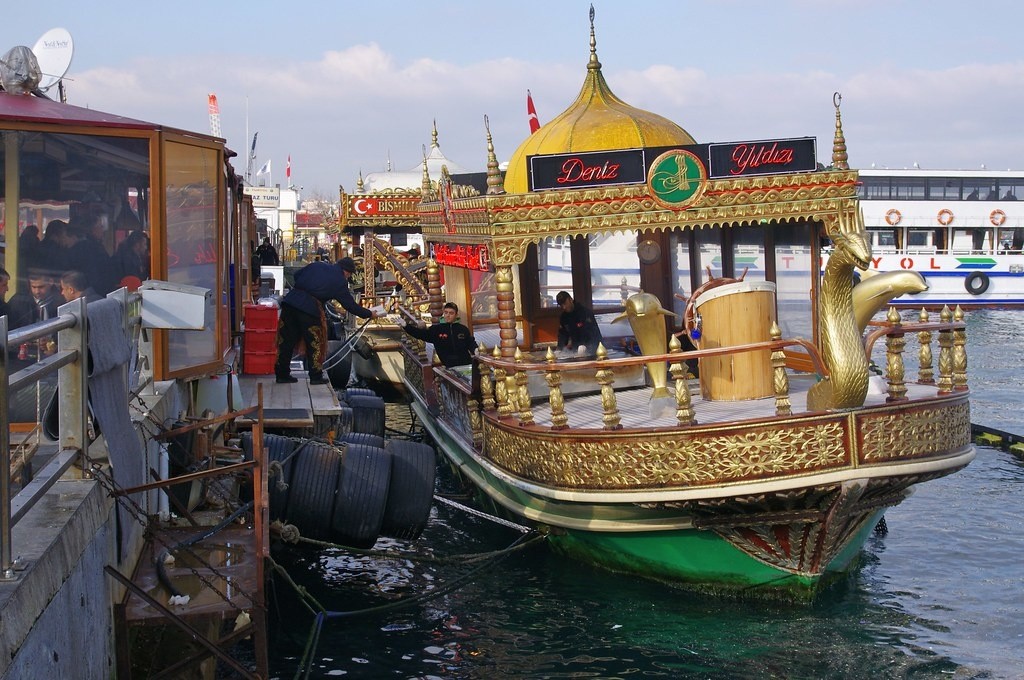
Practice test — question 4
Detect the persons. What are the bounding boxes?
[966,189,1017,201]
[554,290,603,354]
[0,210,418,384]
[392,301,481,367]
[1000,243,1021,255]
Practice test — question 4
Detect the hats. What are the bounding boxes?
[556,291,569,305]
[338,256,357,276]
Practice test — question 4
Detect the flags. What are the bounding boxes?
[528,93,541,134]
[258,158,270,175]
[286,156,291,177]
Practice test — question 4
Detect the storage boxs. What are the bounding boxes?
[244,328,277,353]
[244,305,278,331]
[244,353,277,375]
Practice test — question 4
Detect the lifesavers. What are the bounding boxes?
[379,440,435,539]
[283,444,341,540]
[964,271,990,295]
[349,396,385,437]
[331,443,392,549]
[339,433,385,449]
[937,209,953,225]
[346,386,375,407]
[990,209,1005,225]
[329,340,352,387]
[885,209,901,225]
[331,321,345,340]
[351,337,373,360]
[237,438,291,520]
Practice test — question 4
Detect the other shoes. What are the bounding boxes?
[310,374,329,384]
[276,372,299,383]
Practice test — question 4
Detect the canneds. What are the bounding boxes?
[19,343,27,360]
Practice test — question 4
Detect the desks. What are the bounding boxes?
[7,344,58,420]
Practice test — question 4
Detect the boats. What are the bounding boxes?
[325,4,985,612]
[546,168,1024,308]
